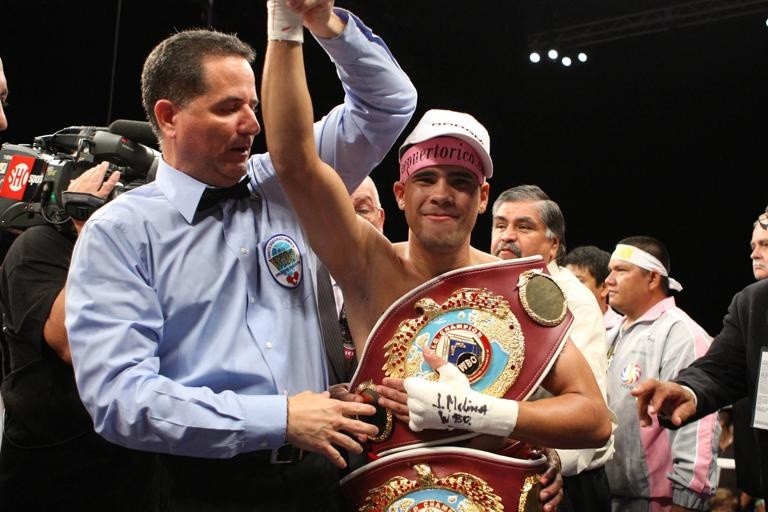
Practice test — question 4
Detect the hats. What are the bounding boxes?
[397,107,497,179]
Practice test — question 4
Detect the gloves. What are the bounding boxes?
[398,361,523,441]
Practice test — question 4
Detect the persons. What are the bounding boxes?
[563,208,768,511]
[3,0,613,512]
